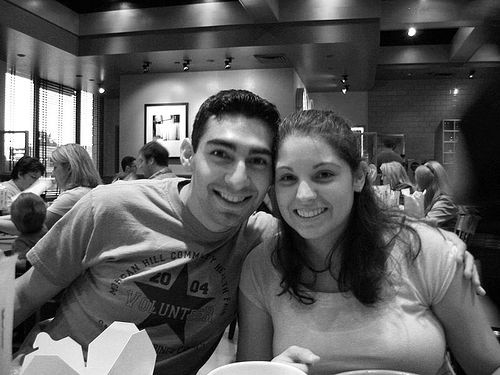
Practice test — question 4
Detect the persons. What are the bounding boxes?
[0,144,105,236]
[414,157,458,233]
[234,108,500,375]
[137,141,181,179]
[0,155,46,215]
[379,160,414,205]
[111,155,138,184]
[10,193,49,281]
[374,138,402,186]
[13,88,486,370]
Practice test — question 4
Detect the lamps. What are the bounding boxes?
[340,77,352,95]
[98,86,110,96]
[140,59,234,71]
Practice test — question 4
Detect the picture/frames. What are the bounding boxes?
[143,102,189,160]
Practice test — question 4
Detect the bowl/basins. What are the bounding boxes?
[207,361,307,375]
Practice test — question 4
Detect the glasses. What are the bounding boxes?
[27,173,39,180]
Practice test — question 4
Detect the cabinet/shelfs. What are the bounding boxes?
[441,118,463,165]
